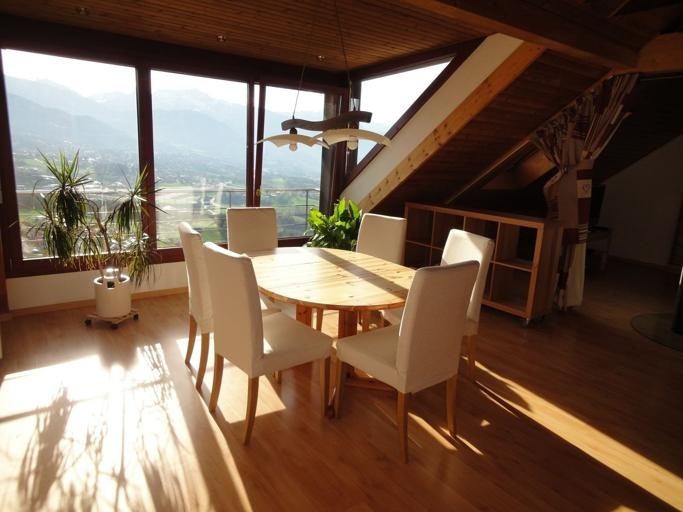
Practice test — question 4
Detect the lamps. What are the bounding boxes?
[256,1,393,151]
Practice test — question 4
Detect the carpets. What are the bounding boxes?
[630,308,682,351]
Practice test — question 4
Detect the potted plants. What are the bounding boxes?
[7,146,168,320]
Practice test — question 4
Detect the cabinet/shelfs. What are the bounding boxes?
[400,200,566,327]
